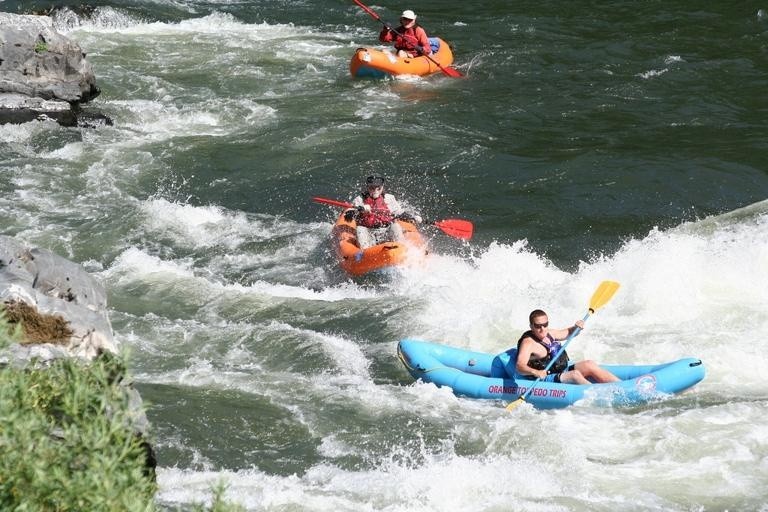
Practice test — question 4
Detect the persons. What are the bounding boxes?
[514,309,623,384]
[380,10,431,57]
[344,173,423,248]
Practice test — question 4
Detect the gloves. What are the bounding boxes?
[361,204,371,214]
[384,22,392,31]
[415,45,422,52]
[410,215,422,224]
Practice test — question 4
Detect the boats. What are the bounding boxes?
[393,337,699,411]
[350,38,454,83]
[330,193,435,290]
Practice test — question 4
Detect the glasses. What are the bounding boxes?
[534,321,548,328]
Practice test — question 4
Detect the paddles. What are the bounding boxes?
[504,280,620,413]
[354,0,465,79]
[314,197,473,240]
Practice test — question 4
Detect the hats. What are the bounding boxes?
[367,173,383,187]
[401,10,415,19]
